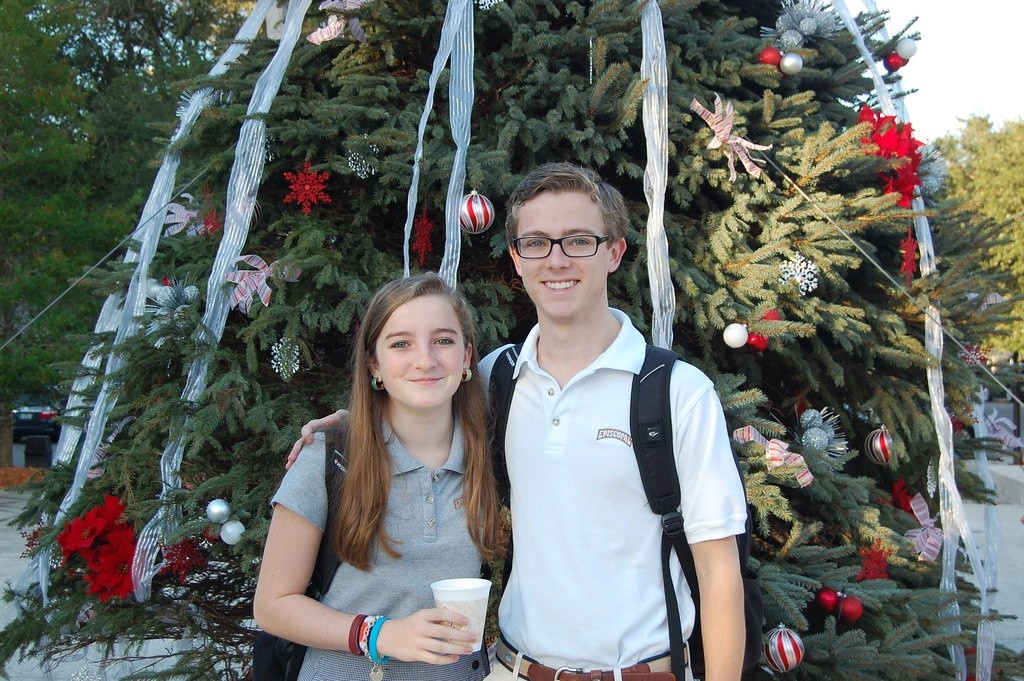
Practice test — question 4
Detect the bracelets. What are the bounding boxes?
[348,613,392,681]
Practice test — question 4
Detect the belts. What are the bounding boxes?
[490,635,691,680]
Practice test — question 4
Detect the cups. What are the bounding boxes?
[430,578,492,651]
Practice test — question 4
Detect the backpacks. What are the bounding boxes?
[487,341,762,680]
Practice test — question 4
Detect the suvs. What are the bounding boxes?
[12,388,63,444]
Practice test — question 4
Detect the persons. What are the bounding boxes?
[253,273,502,681]
[285,160,748,681]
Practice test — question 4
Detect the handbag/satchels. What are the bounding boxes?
[247,425,355,681]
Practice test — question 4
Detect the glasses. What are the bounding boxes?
[511,234,610,260]
